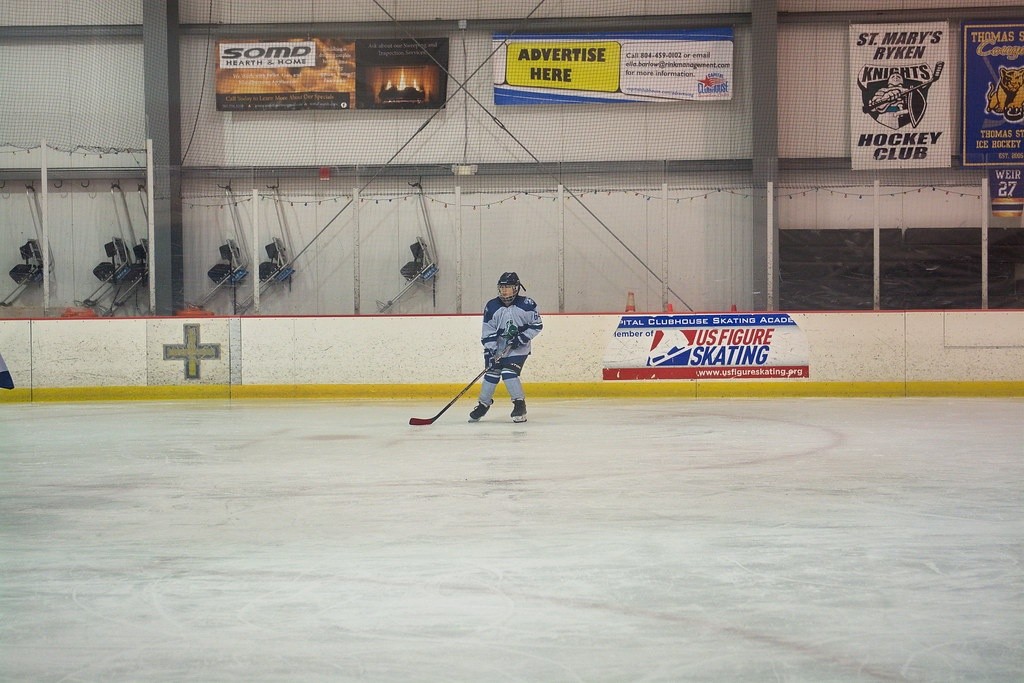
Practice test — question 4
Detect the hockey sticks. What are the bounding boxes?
[410,343,513,425]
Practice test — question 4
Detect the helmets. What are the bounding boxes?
[497,272,520,294]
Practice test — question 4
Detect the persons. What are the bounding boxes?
[468,272,543,423]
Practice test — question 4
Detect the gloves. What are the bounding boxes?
[489,348,501,371]
[506,332,530,350]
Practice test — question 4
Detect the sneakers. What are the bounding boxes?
[510,398,527,423]
[468,401,490,423]
